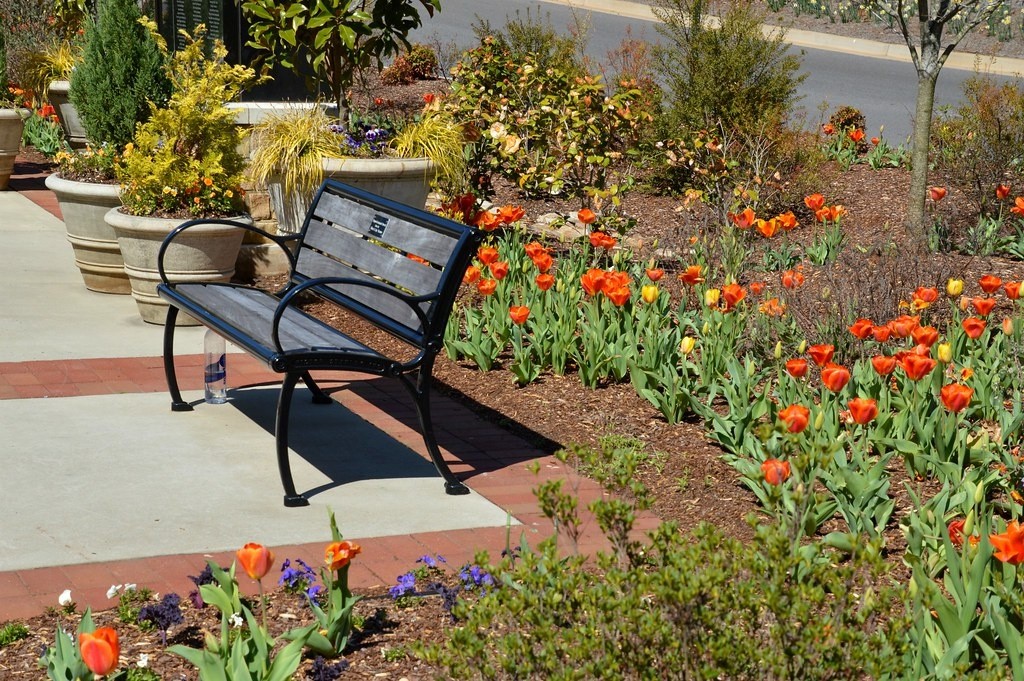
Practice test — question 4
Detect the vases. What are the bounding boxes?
[45,171,131,295]
[0,109,32,189]
[105,206,253,327]
[51,80,91,144]
[269,156,443,240]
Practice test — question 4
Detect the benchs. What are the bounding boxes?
[162,179,484,506]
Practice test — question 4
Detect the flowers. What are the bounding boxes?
[0,0,87,109]
[108,23,275,221]
[53,1,179,181]
[248,100,476,211]
